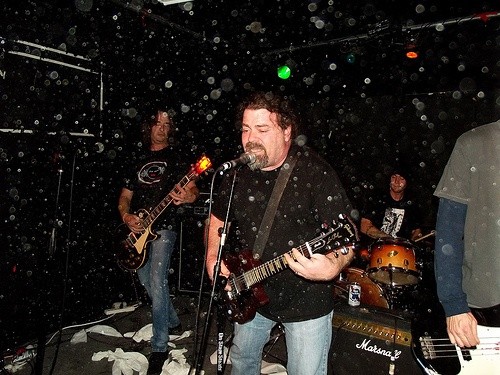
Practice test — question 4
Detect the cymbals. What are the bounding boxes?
[413,201,440,247]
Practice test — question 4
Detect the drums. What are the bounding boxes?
[368,237,421,286]
[334,267,393,310]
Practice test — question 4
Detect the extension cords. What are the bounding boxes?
[104,304,139,315]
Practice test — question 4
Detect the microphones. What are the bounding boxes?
[218,152,257,171]
[77,142,104,153]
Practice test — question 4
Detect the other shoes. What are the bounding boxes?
[168,323,182,334]
[147,349,169,375]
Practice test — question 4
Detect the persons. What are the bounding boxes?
[118,106,197,375]
[206,92,355,375]
[434,120,500,375]
[361,167,429,291]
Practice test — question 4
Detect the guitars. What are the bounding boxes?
[411,295,500,375]
[111,153,212,270]
[215,214,361,325]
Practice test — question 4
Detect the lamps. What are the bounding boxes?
[404,31,419,60]
[347,47,361,63]
[277,59,296,79]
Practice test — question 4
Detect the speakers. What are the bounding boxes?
[177,220,217,296]
[327,300,430,375]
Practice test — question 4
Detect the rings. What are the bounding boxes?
[295,270,298,275]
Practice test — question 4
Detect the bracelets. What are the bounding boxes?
[121,213,128,223]
